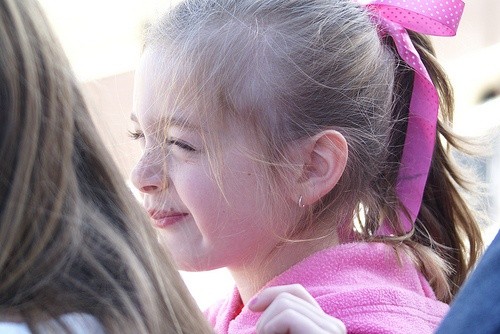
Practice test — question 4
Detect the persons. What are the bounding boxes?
[127,0,500,334]
[0,0,213,334]
